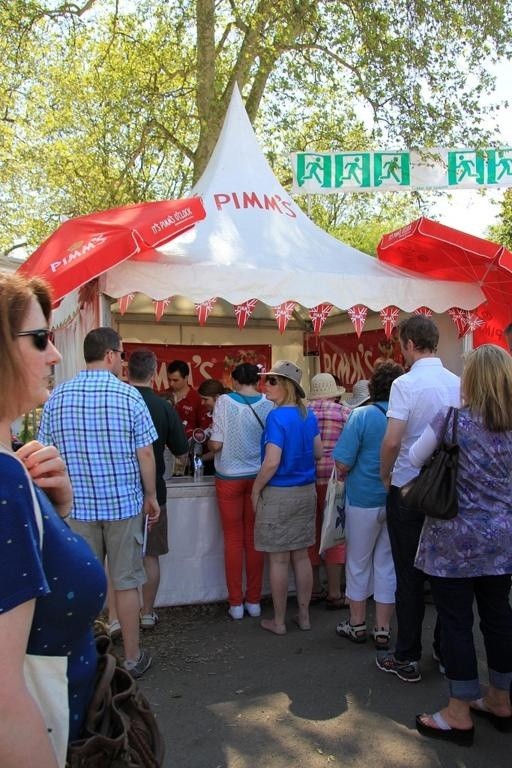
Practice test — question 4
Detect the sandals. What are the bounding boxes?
[337,618,367,641]
[372,627,392,650]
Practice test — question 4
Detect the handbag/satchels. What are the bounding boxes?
[318,465,347,555]
[67,635,166,767]
[396,442,459,520]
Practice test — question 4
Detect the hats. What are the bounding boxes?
[256,359,306,399]
[341,380,371,408]
[307,373,345,400]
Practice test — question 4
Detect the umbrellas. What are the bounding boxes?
[378,216,511,355]
[14,193,206,310]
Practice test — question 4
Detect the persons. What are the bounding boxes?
[0,270,107,768]
[331,357,407,649]
[39,327,160,679]
[374,314,467,683]
[104,347,188,640]
[250,361,325,635]
[207,364,276,620]
[161,359,236,463]
[300,370,356,607]
[413,343,512,747]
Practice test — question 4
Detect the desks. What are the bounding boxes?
[102,476,328,611]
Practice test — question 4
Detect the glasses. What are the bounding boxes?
[264,375,278,385]
[14,328,59,351]
[106,349,128,361]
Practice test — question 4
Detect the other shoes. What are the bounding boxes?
[261,619,286,635]
[231,601,260,619]
[294,614,311,630]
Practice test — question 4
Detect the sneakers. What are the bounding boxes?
[93,608,158,678]
[432,649,446,677]
[376,653,422,681]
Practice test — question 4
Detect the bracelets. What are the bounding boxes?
[63,514,70,522]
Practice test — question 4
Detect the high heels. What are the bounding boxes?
[417,712,475,745]
[470,697,510,733]
